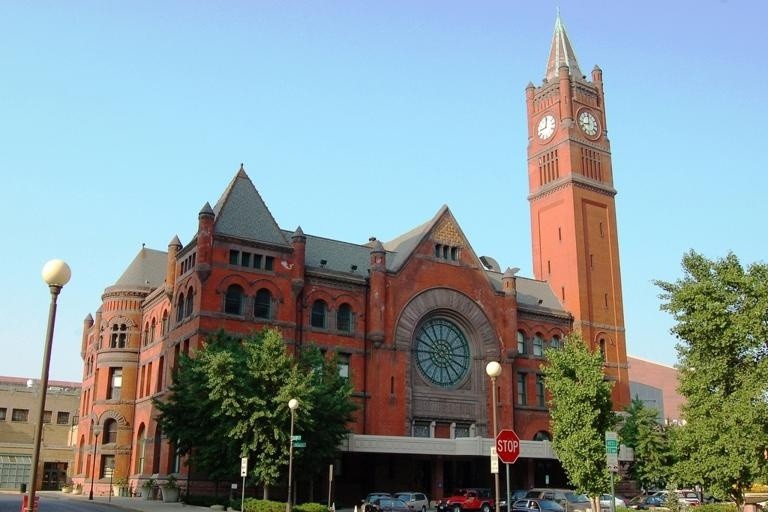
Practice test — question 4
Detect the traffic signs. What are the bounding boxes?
[291,436,302,440]
[294,442,306,447]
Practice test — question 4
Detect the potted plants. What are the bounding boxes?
[72,483,83,494]
[60,480,75,493]
[159,475,181,503]
[111,477,129,497]
[140,477,160,501]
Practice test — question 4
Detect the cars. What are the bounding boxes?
[362,492,394,511]
[363,498,415,512]
[575,493,627,512]
[499,490,528,512]
[628,489,701,508]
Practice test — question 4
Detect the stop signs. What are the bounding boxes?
[495,427,521,463]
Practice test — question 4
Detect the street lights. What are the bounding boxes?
[285,399,301,512]
[485,361,501,511]
[25,260,73,511]
[88,428,102,500]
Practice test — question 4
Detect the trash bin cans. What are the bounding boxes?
[20,484,26,493]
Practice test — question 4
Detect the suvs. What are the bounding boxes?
[392,491,430,512]
[513,487,591,512]
[434,487,494,512]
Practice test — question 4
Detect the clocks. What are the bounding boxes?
[536,113,555,141]
[578,111,600,137]
